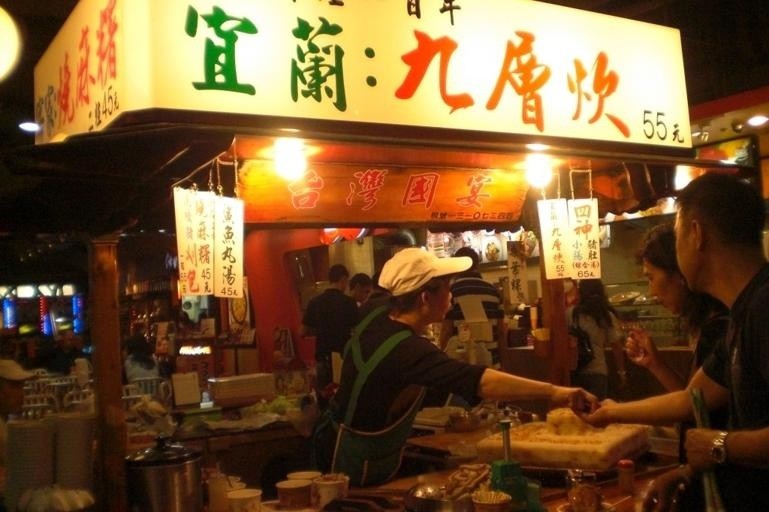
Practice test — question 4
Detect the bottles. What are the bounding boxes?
[618,460,635,493]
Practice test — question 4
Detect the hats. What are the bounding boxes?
[377,248,473,299]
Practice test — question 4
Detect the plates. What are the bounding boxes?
[609,291,640,306]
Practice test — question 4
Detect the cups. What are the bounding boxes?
[471,490,512,512]
[275,470,350,509]
[566,476,602,512]
[194,468,262,512]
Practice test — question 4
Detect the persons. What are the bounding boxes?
[566,276,629,399]
[0,321,173,388]
[346,273,372,305]
[300,262,357,393]
[570,172,768,510]
[622,219,732,512]
[440,246,507,413]
[313,246,596,491]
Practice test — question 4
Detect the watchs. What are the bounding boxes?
[709,430,728,464]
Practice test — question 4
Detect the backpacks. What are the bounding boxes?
[568,324,593,371]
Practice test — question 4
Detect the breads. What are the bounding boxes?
[476,407,647,472]
